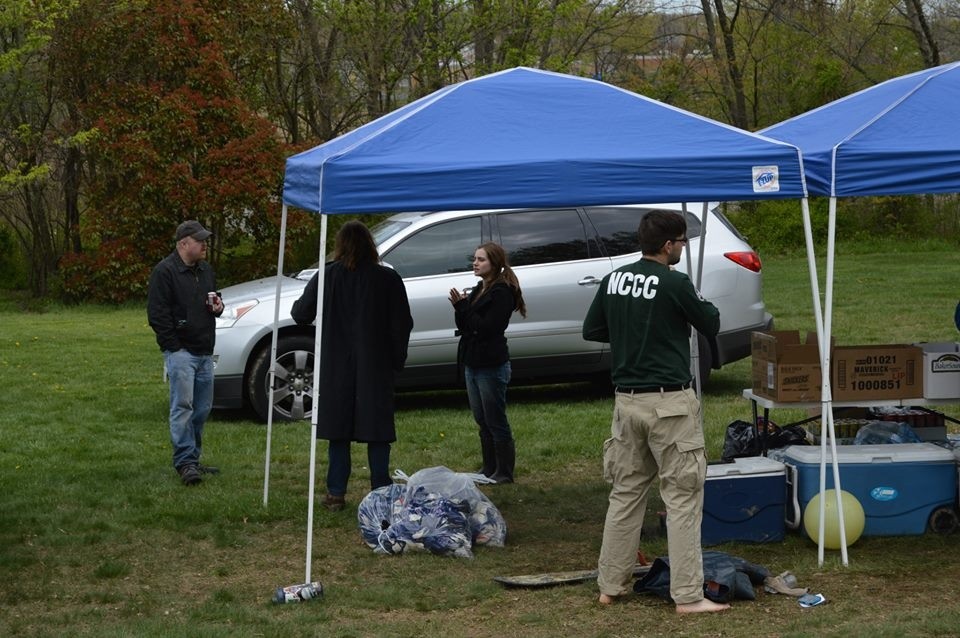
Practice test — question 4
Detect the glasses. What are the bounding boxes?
[660,237,688,249]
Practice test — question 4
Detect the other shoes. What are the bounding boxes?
[322,493,346,509]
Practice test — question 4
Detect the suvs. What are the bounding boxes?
[162,197,777,424]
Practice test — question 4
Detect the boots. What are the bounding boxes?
[484,442,515,484]
[477,441,496,477]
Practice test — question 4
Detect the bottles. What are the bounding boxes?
[272,581,323,604]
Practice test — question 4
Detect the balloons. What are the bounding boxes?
[804,489,865,550]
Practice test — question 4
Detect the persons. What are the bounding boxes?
[581,212,730,612]
[146,221,225,483]
[448,243,527,484]
[290,221,414,509]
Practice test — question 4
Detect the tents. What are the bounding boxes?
[691,64,960,565]
[263,66,848,584]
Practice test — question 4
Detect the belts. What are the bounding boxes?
[616,385,689,393]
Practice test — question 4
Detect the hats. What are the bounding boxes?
[176,220,212,240]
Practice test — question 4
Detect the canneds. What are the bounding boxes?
[208,292,218,313]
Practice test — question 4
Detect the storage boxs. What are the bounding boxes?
[805,427,947,445]
[699,456,788,545]
[831,344,924,400]
[750,330,837,403]
[912,343,960,399]
[784,445,960,540]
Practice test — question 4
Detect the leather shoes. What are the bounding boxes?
[198,466,222,475]
[182,466,202,484]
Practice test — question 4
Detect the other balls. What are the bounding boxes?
[804,489,865,550]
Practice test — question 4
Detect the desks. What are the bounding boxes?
[743,388,960,457]
